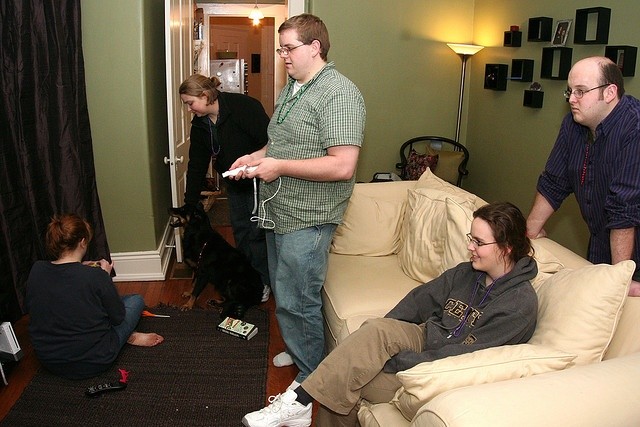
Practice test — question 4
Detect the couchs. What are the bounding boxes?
[321,181,640,426]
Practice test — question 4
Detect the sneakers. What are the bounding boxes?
[261,283,271,302]
[242,390,313,427]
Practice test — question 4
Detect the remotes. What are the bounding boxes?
[86,382,125,396]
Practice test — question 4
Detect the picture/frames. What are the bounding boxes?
[551,19,572,47]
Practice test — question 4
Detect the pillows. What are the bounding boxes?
[416,167,476,204]
[328,193,405,256]
[389,344,578,422]
[399,189,477,284]
[405,149,439,181]
[437,197,563,279]
[425,142,465,187]
[529,260,636,369]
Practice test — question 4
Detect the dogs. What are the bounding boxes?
[167,200,265,319]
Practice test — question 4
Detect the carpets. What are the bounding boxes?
[0,303,269,427]
[201,199,233,226]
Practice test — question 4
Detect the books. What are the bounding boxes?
[216,315,259,341]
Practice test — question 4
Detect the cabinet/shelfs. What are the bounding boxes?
[523,90,544,108]
[510,59,534,82]
[540,46,573,80]
[194,39,204,45]
[504,31,522,47]
[605,45,638,77]
[575,7,611,44]
[484,64,508,91]
[527,17,553,42]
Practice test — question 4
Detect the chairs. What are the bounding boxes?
[397,136,470,188]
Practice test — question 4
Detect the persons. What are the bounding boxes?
[23,213,166,379]
[178,72,270,302]
[526,54,640,297]
[227,13,367,395]
[241,200,539,427]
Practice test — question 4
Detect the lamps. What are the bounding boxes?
[446,43,485,153]
[248,0,264,26]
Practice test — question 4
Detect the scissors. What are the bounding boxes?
[140,310,171,318]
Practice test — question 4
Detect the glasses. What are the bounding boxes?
[467,233,498,250]
[276,41,312,55]
[564,84,611,98]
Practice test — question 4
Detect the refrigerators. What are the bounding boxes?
[210,59,249,95]
